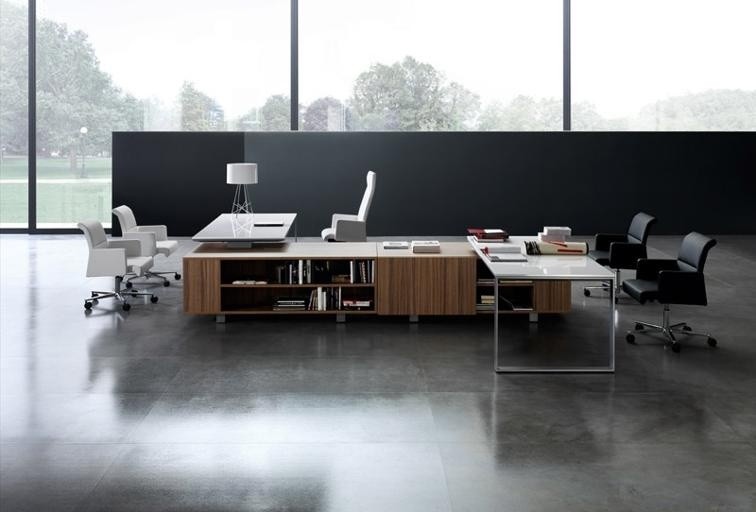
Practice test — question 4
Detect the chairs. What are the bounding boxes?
[112,205,181,287]
[583,211,656,303]
[321,171,376,242]
[623,232,718,353]
[77,218,159,311]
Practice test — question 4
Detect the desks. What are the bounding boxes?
[466,235,616,373]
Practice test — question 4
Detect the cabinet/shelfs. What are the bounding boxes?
[183,242,572,324]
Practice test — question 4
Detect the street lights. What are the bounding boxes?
[76,126,89,179]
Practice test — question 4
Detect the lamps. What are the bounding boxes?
[226,162,258,213]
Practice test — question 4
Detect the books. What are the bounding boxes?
[268,260,374,311]
[481,295,495,304]
[383,240,441,253]
[473,226,589,262]
